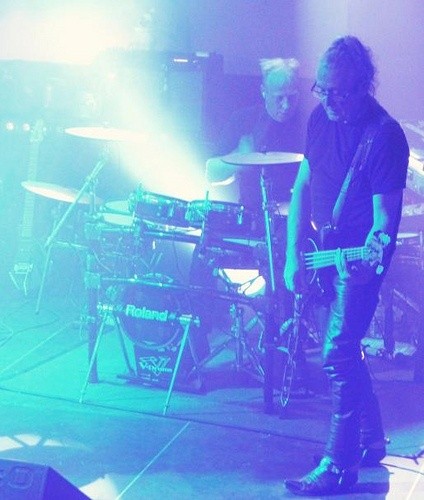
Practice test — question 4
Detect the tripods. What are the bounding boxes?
[187,304,265,382]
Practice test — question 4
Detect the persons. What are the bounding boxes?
[284,37,410,496]
[190,58,307,365]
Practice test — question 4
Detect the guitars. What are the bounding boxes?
[1,118,46,299]
[302,229,393,299]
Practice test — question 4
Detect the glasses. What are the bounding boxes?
[311,80,356,102]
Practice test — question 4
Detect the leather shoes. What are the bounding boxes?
[285,457,358,495]
[314,446,386,465]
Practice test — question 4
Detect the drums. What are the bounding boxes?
[184,200,260,234]
[102,200,135,216]
[92,212,157,253]
[128,189,190,228]
[116,272,192,350]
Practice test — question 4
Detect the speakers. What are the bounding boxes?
[0,459,92,500]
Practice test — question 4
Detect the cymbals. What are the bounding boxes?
[21,181,103,204]
[219,151,304,166]
[63,126,130,141]
